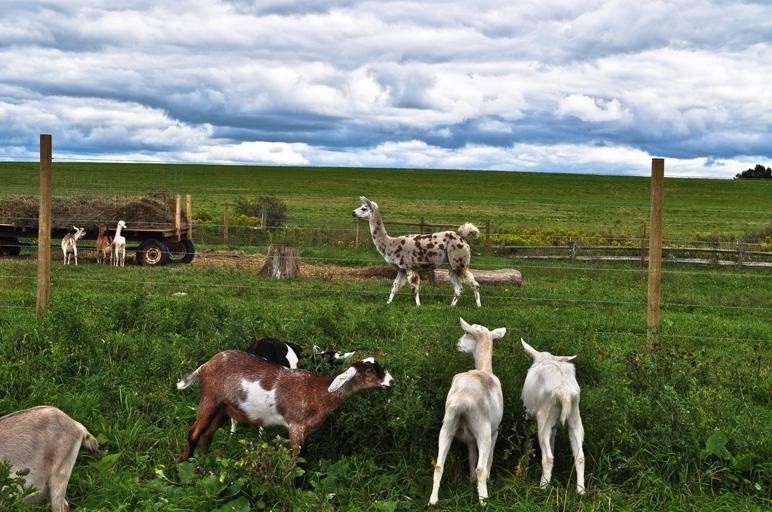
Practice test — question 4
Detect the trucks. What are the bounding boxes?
[0,217,197,267]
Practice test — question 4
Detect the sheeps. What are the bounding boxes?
[95,219,128,269]
[172,337,396,490]
[0,404,100,512]
[61,225,87,266]
[520,337,586,498]
[425,315,507,511]
